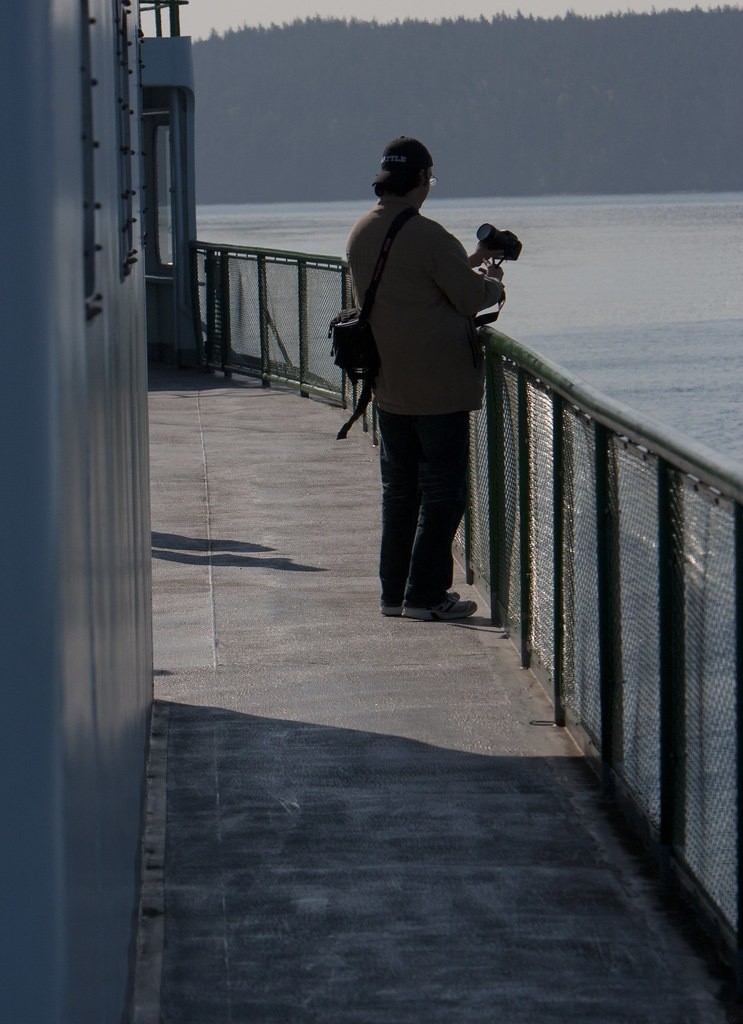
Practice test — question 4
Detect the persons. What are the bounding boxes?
[346,137,506,619]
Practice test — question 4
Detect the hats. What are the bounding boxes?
[371,135,434,196]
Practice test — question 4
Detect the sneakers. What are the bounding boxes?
[380,592,478,621]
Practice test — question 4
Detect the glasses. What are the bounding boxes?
[429,174,438,187]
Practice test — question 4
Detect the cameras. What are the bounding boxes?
[477,224,522,261]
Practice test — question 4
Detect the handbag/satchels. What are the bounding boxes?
[326,307,381,442]
